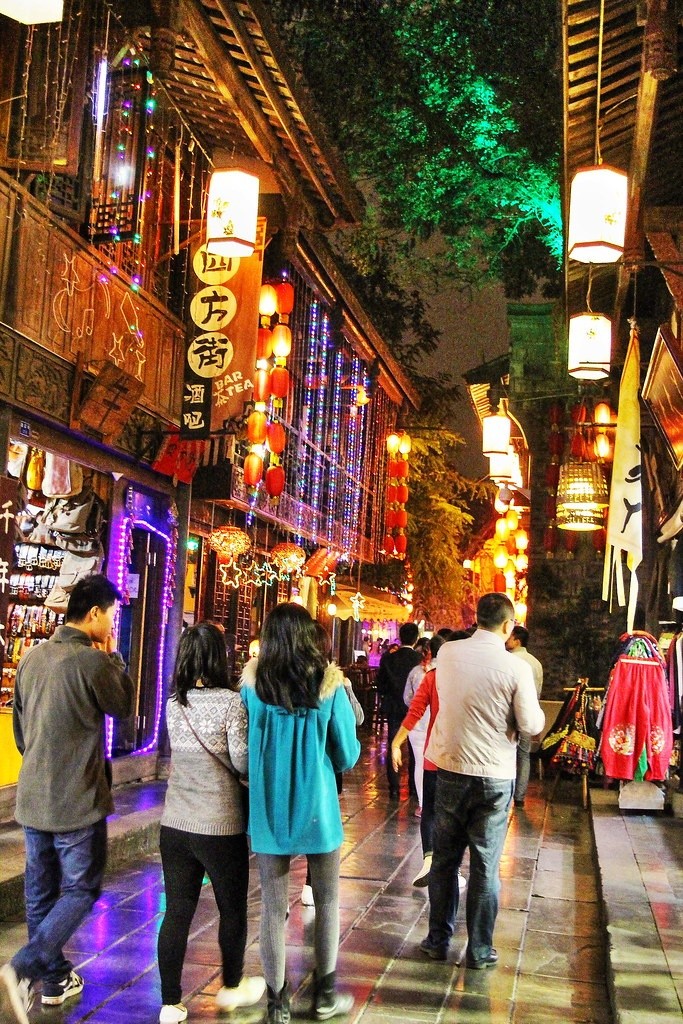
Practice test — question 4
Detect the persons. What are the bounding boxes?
[417,592,546,969]
[239,602,364,1024]
[157,620,266,1024]
[0,574,134,1024]
[353,621,544,887]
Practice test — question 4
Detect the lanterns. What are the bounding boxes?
[210,526,251,565]
[481,416,523,491]
[384,432,411,558]
[566,165,632,263]
[246,281,292,499]
[490,486,530,595]
[206,167,260,258]
[272,543,306,574]
[568,312,612,380]
[544,401,616,559]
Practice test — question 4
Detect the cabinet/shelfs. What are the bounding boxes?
[0,543,65,715]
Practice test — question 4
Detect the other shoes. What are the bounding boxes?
[458,875,466,889]
[414,807,422,818]
[302,884,314,905]
[159,1002,188,1024]
[216,977,266,1013]
[411,788,416,795]
[412,863,432,887]
[389,788,399,798]
[515,800,524,806]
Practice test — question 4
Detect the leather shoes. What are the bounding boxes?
[468,948,498,969]
[420,939,447,959]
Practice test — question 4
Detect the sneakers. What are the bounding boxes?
[0,964,36,1024]
[41,971,84,1005]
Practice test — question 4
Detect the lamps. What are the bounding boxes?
[563,117,629,264]
[207,168,258,257]
[482,413,524,489]
[568,265,611,382]
[555,463,609,531]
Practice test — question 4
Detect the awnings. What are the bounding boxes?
[331,589,409,623]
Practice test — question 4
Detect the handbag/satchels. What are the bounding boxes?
[27,487,104,615]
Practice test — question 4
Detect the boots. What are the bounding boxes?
[311,967,354,1020]
[266,979,291,1024]
